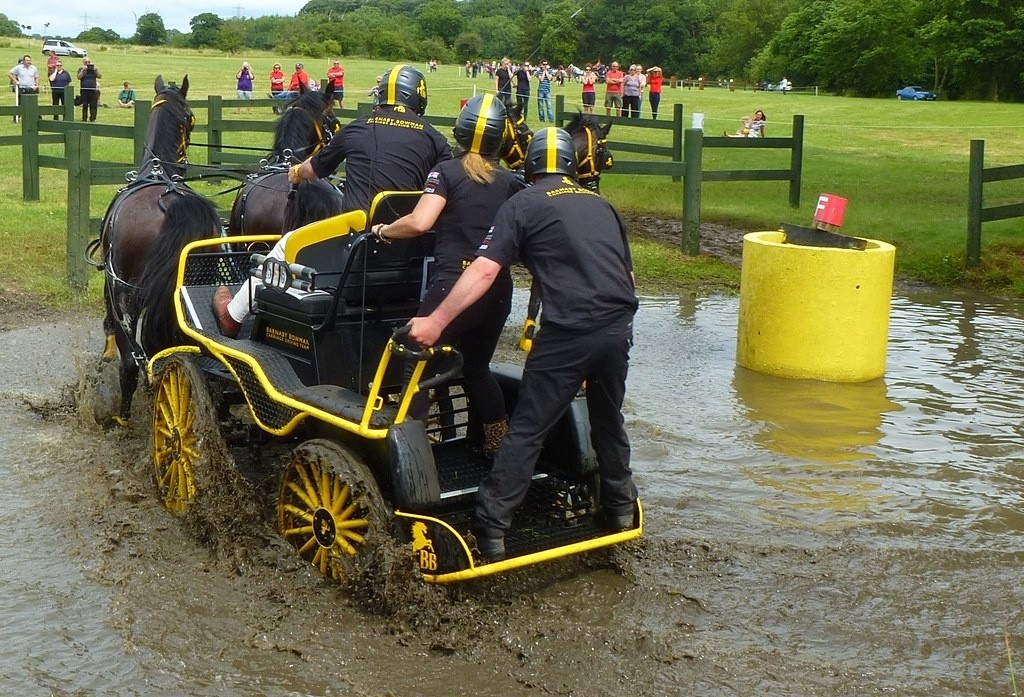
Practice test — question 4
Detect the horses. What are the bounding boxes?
[97,75,224,428]
[226,77,342,274]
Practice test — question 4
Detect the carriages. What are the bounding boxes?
[82,73,643,583]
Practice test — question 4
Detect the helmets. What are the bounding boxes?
[378,64,427,117]
[453,93,508,154]
[525,127,577,179]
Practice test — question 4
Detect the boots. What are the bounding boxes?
[468,415,510,468]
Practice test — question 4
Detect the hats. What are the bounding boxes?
[124,81,129,86]
[296,63,303,69]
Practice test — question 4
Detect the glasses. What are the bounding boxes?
[613,66,618,67]
[334,63,339,65]
[525,65,529,67]
[585,69,591,71]
[274,67,281,69]
[56,64,62,66]
[543,64,547,65]
[84,61,90,63]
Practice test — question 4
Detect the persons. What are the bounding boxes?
[6,55,42,119]
[326,61,346,109]
[269,63,285,113]
[76,57,102,123]
[213,65,453,338]
[48,60,72,121]
[535,59,554,123]
[781,76,788,95]
[269,63,310,99]
[509,62,538,75]
[723,110,766,138]
[117,82,136,108]
[466,61,498,79]
[556,61,663,118]
[511,61,533,121]
[235,61,256,114]
[429,60,437,73]
[406,127,639,554]
[494,58,514,107]
[47,50,60,77]
[370,93,527,463]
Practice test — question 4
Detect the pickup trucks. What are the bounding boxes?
[896,86,937,101]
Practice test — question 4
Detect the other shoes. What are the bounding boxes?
[540,119,545,122]
[212,286,242,338]
[475,537,505,561]
[724,130,727,137]
[593,511,634,532]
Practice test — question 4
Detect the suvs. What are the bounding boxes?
[42,40,87,58]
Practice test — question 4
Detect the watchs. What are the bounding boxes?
[293,164,304,180]
